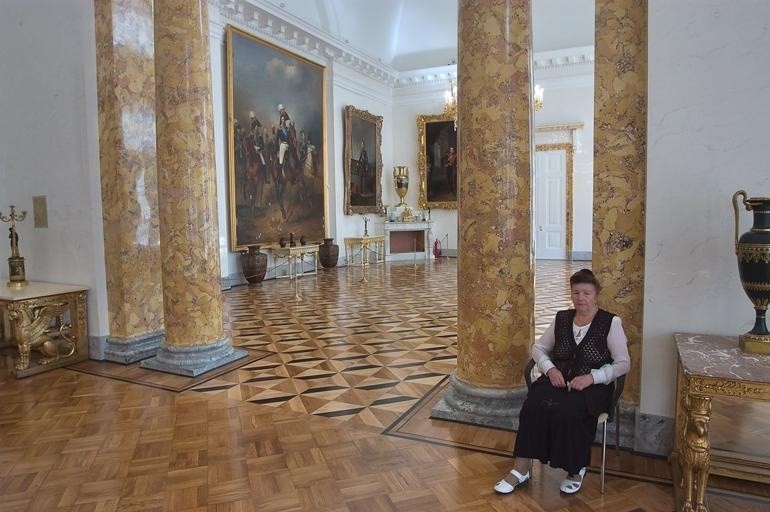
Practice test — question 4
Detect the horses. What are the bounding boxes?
[235,123,318,223]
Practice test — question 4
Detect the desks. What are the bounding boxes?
[669,328,769,511]
[269,243,320,284]
[0,277,89,380]
[343,234,385,267]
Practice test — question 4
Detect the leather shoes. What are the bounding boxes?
[559,465,587,495]
[493,468,530,495]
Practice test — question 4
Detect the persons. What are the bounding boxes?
[234,103,317,187]
[493,269,632,494]
[357,141,368,194]
[443,147,457,197]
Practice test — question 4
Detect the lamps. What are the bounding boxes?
[439,76,547,125]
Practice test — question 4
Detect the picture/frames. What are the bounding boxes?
[339,103,389,217]
[218,21,332,256]
[413,97,461,212]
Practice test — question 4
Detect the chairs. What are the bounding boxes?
[521,350,628,495]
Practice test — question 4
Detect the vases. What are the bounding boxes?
[317,237,340,269]
[237,244,268,288]
[728,187,770,358]
